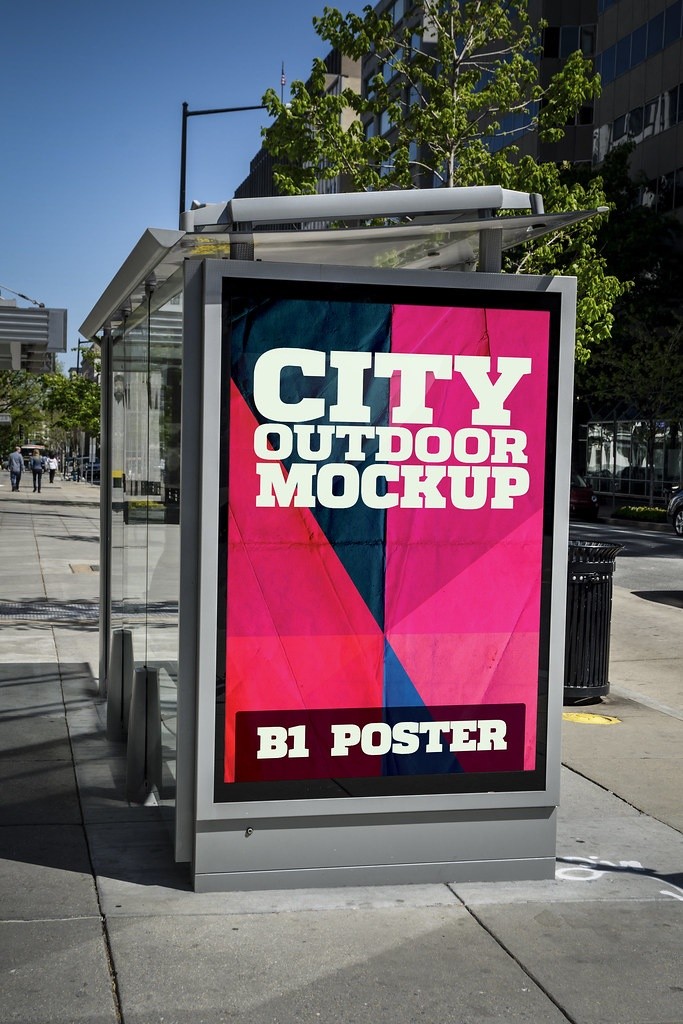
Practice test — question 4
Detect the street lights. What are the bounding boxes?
[180,100,294,212]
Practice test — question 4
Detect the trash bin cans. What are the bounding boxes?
[564,536,628,708]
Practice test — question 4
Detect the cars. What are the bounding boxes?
[584,466,680,507]
[570,473,600,523]
[28,457,101,481]
[666,487,683,537]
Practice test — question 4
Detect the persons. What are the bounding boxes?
[30,450,45,493]
[7,446,25,493]
[46,453,58,484]
